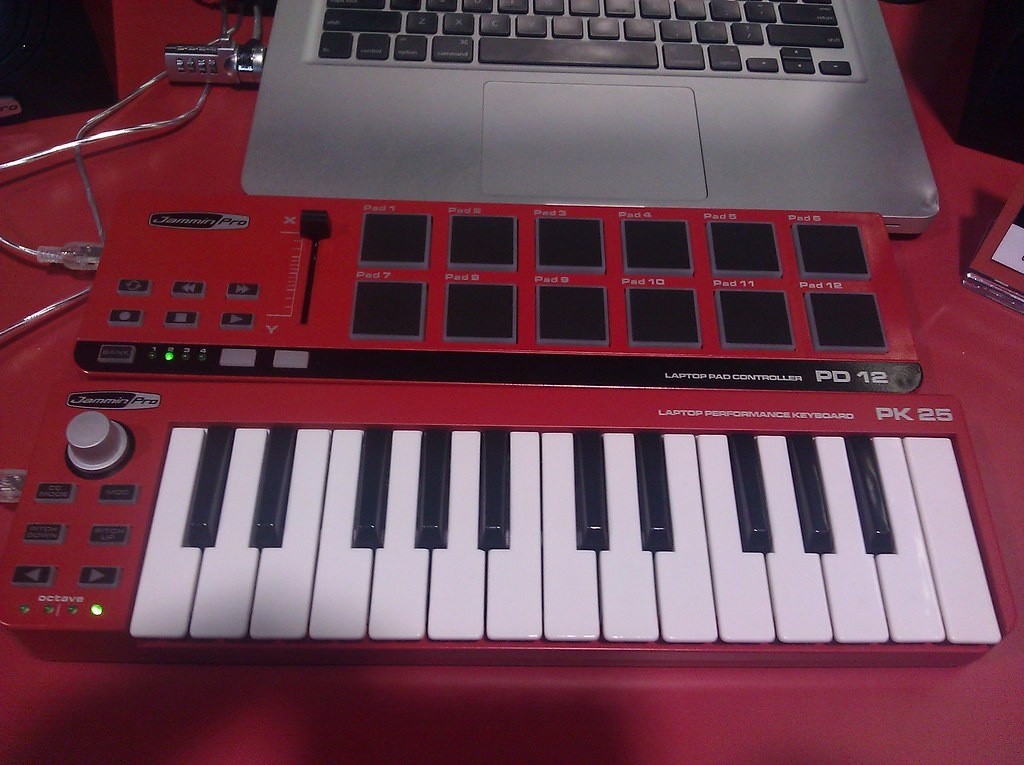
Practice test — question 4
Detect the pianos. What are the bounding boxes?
[0,375,1024,667]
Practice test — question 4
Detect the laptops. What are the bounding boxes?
[241,0,940,235]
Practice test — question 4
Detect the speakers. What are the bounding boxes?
[0,0,118,126]
[957,0,1024,166]
